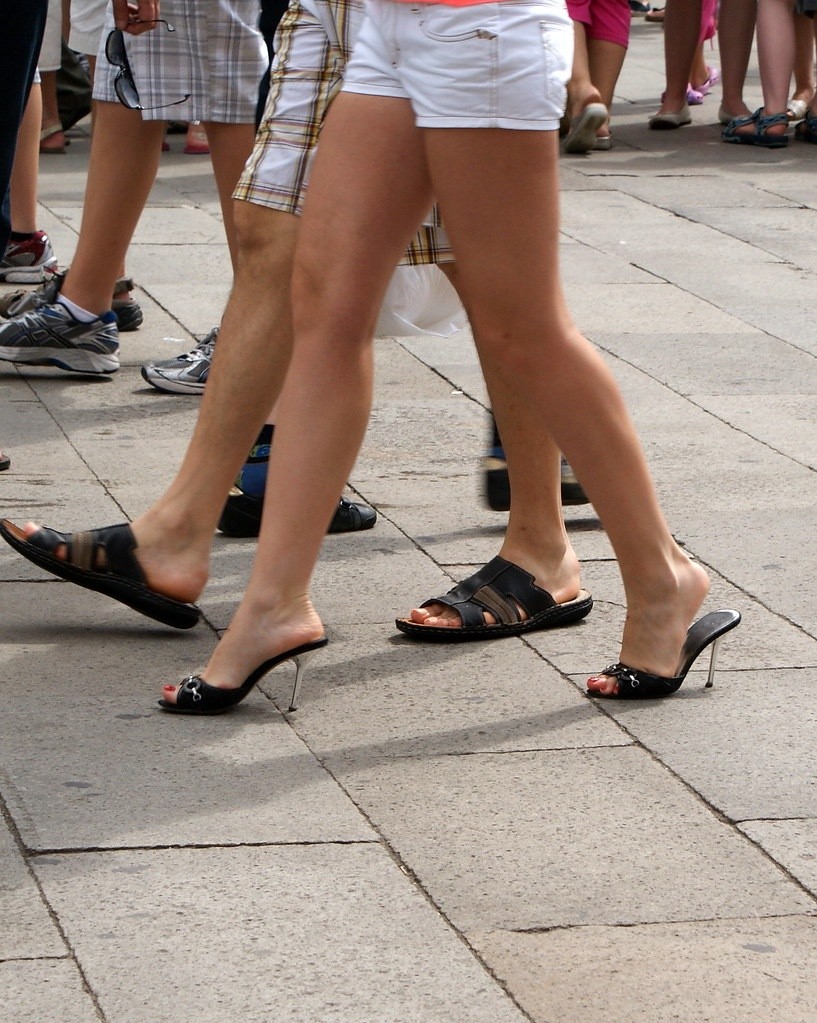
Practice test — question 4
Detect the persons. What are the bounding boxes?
[0,0,269,394]
[720,0,817,147]
[38,0,110,154]
[0,0,58,286]
[661,0,718,104]
[0,0,144,327]
[162,121,209,154]
[0,0,594,642]
[559,0,632,153]
[628,0,666,22]
[786,0,817,121]
[156,0,740,716]
[647,0,758,131]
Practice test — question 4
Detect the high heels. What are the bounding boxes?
[152,634,331,715]
[586,605,743,702]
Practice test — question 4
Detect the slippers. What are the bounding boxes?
[562,102,614,154]
[0,517,201,631]
[660,65,720,105]
[786,98,810,120]
[395,555,596,639]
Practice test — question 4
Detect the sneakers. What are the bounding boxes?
[141,326,227,394]
[482,456,599,512]
[217,482,379,541]
[2,228,59,283]
[0,279,120,374]
[1,263,144,331]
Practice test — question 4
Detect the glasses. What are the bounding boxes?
[105,18,191,110]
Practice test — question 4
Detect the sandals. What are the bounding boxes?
[721,106,816,149]
[39,124,67,155]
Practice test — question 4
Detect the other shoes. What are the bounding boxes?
[648,100,694,132]
[718,104,753,123]
[185,119,209,153]
